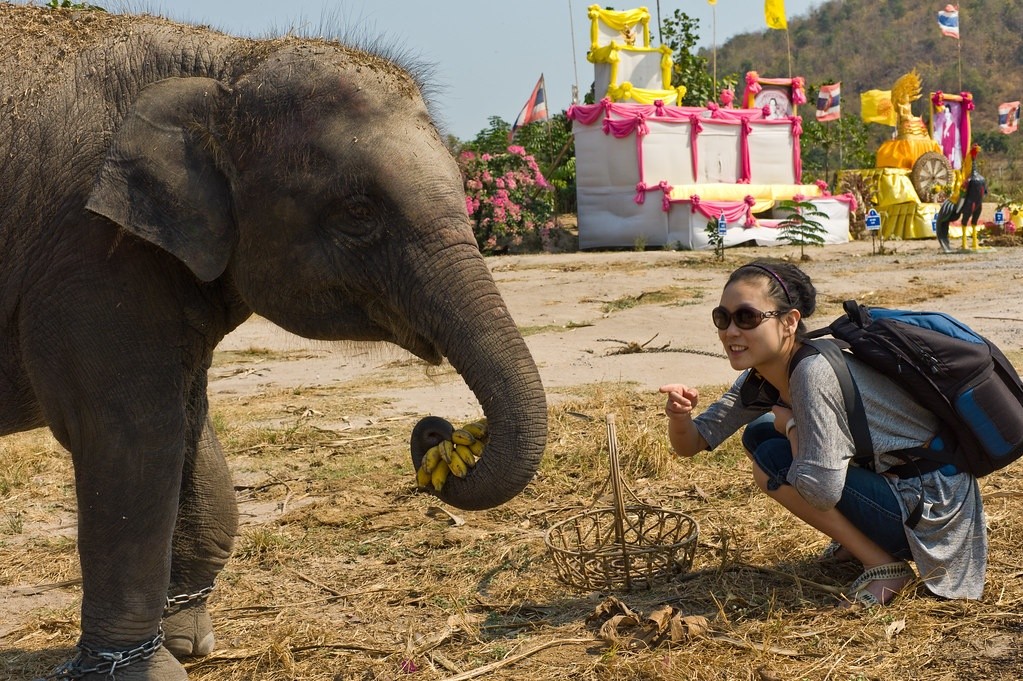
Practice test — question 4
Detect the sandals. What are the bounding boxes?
[818,539,863,567]
[837,559,917,612]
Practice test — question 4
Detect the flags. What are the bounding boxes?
[508,76,546,141]
[816,84,840,122]
[998,101,1021,133]
[938,5,958,39]
[764,0,787,30]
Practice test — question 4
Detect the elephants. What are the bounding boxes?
[0,0,550,680]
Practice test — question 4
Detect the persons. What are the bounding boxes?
[659,258,987,611]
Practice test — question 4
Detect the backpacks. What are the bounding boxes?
[712,307,790,329]
[738,300,1023,480]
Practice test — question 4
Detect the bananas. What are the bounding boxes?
[415,416,489,492]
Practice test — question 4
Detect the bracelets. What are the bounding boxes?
[786,418,795,437]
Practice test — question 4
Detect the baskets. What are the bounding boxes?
[544,413,699,592]
